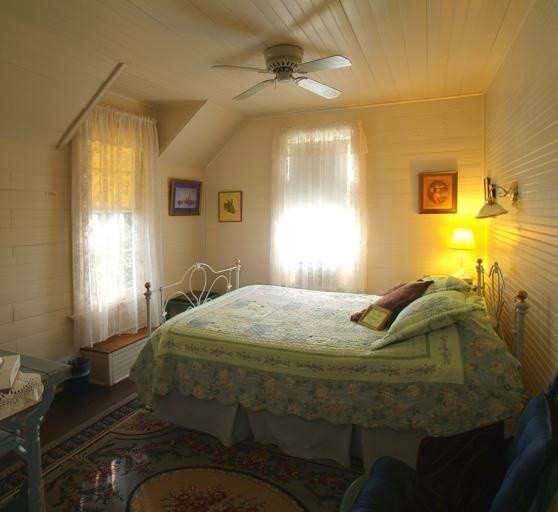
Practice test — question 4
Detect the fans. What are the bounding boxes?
[207,43,354,106]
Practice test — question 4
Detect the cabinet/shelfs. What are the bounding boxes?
[165,289,221,321]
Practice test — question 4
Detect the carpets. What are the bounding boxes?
[2,390,385,511]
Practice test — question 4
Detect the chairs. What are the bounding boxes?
[348,389,558,512]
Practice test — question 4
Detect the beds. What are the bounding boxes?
[124,257,530,470]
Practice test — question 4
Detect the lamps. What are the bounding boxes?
[447,226,477,280]
[475,177,519,220]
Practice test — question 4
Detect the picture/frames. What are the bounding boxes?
[218,191,242,222]
[359,303,392,331]
[417,172,458,214]
[170,178,202,216]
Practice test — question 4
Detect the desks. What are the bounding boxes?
[0,347,70,512]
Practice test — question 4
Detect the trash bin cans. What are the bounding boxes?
[64,357,92,397]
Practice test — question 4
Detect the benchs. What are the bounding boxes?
[80,325,159,388]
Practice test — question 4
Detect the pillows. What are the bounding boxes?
[375,280,407,297]
[416,275,476,297]
[369,288,483,351]
[348,279,434,321]
[400,418,509,511]
